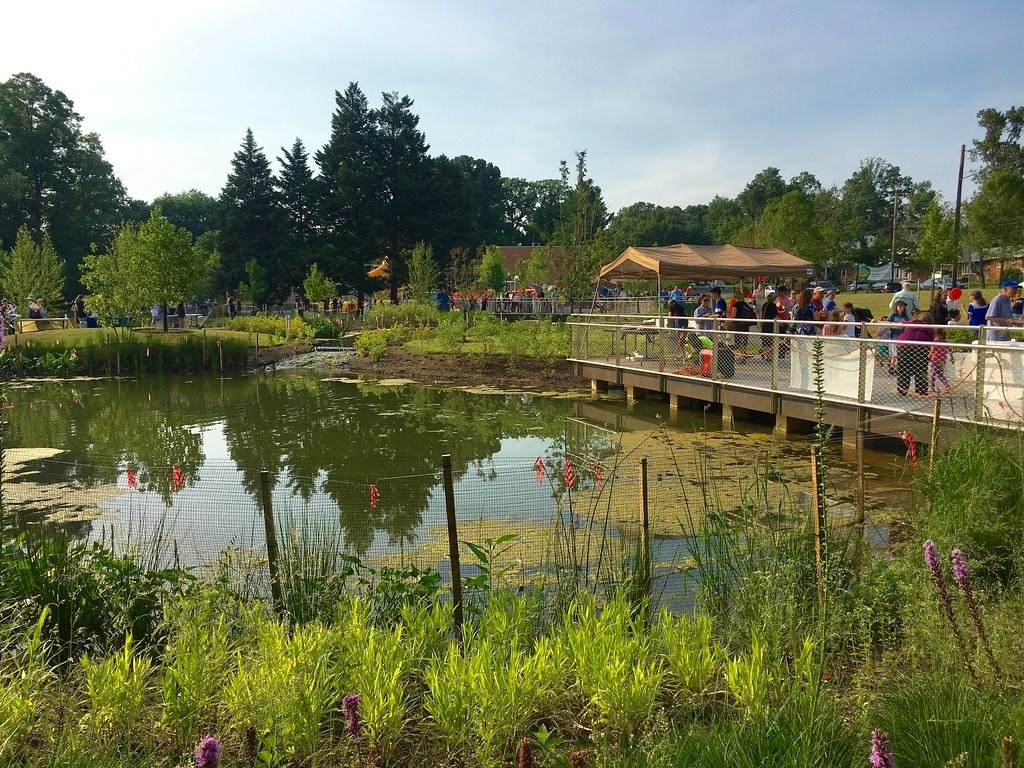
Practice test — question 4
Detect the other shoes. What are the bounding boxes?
[759,348,766,359]
[735,357,746,365]
[940,386,955,395]
[927,388,937,392]
[888,367,898,376]
[766,356,773,362]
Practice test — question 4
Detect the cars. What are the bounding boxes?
[847,281,870,291]
[708,280,726,290]
[809,280,840,295]
[765,285,788,297]
[920,276,968,291]
[867,282,902,293]
[900,279,921,291]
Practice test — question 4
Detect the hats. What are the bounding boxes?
[826,290,837,296]
[896,300,907,306]
[903,282,910,284]
[26,293,36,299]
[1002,280,1023,289]
[968,290,982,295]
[814,287,825,293]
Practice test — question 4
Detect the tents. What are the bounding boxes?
[580,244,820,364]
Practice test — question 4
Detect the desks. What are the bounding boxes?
[14,317,70,334]
[167,313,203,328]
[602,328,659,367]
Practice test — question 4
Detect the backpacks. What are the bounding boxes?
[742,305,757,326]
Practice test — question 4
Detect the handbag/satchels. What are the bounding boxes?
[29,302,40,312]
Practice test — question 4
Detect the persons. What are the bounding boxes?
[0,280,1024,397]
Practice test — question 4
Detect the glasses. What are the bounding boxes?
[791,293,796,295]
[702,299,709,302]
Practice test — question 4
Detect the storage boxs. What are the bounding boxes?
[700,346,735,378]
